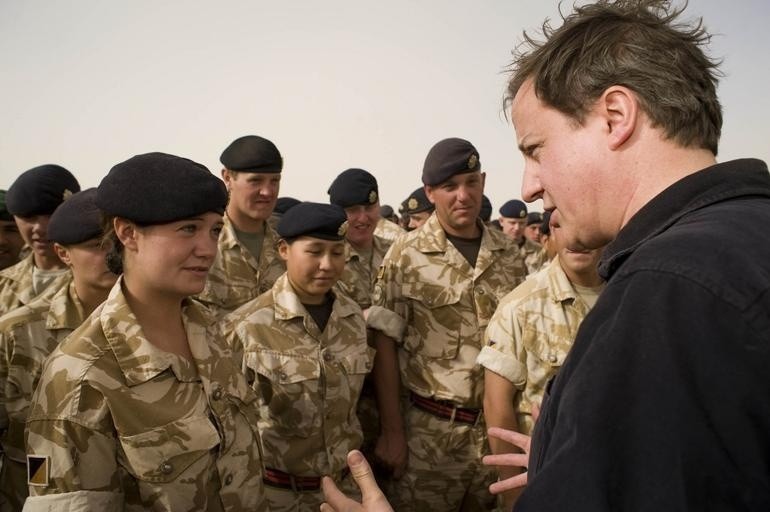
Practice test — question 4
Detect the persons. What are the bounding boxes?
[0,162,81,318]
[498,199,542,261]
[319,0,770,512]
[0,190,27,271]
[327,168,409,442]
[218,200,377,512]
[22,152,270,512]
[192,135,288,322]
[0,187,120,512]
[364,137,528,512]
[402,186,435,229]
[524,211,544,243]
[476,219,607,512]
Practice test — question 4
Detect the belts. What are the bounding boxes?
[409,391,479,424]
[262,465,350,488]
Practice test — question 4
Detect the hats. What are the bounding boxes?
[6,165,81,216]
[398,199,408,213]
[422,138,480,186]
[541,212,551,235]
[220,135,283,173]
[526,213,542,226]
[490,220,503,230]
[0,209,15,221]
[409,187,435,213]
[327,168,378,206]
[273,197,301,213]
[46,186,106,245]
[479,194,493,223]
[276,202,349,241]
[499,200,527,218]
[380,205,394,218]
[95,152,228,225]
